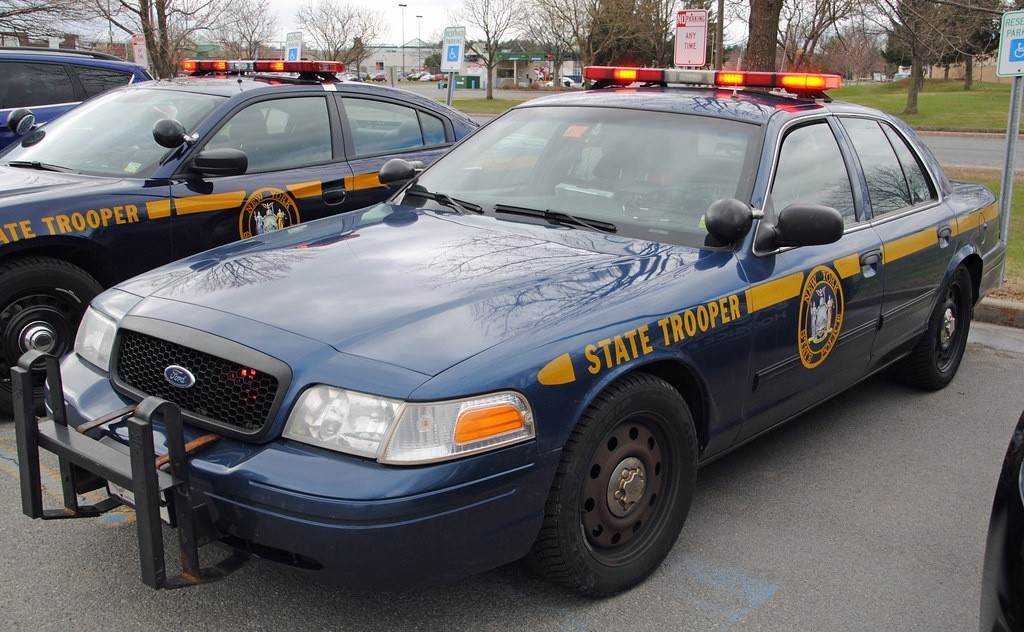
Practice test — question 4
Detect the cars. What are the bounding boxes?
[397,71,448,82]
[546,76,574,88]
[336,69,387,82]
[8,65,1007,601]
[0,55,483,425]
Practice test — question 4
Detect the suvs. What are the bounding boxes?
[1,46,155,154]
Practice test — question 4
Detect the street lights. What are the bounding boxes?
[416,15,423,72]
[398,3,408,78]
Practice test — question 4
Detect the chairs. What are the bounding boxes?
[229,110,326,169]
